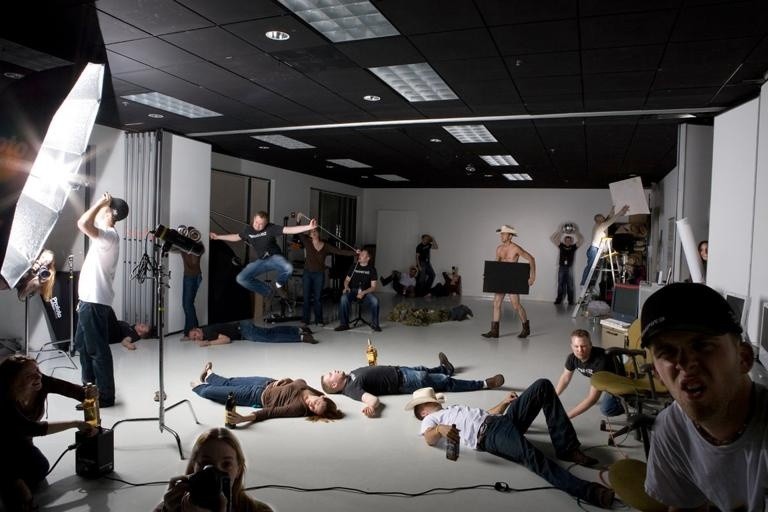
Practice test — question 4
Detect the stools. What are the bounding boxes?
[347,299,370,328]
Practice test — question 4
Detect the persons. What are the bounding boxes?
[380,266,418,295]
[185,320,318,347]
[550,222,585,305]
[208,211,318,305]
[405,377,615,509]
[189,361,343,424]
[291,208,360,328]
[555,330,630,420]
[151,428,274,509]
[74,190,130,408]
[414,234,439,297]
[579,203,630,290]
[0,352,100,512]
[424,271,462,297]
[640,282,768,511]
[170,226,205,342]
[1,248,56,305]
[320,343,504,418]
[687,240,709,284]
[481,224,535,338]
[109,320,154,350]
[335,249,382,331]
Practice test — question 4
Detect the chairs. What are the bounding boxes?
[590,345,673,461]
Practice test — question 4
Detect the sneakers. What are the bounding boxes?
[554,301,563,304]
[298,321,326,344]
[263,289,274,303]
[335,325,349,331]
[568,301,575,305]
[380,276,387,287]
[559,450,597,466]
[200,363,212,381]
[275,286,288,297]
[370,323,382,333]
[439,352,455,376]
[484,374,504,389]
[584,484,615,508]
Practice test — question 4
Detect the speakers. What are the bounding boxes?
[75,427,114,479]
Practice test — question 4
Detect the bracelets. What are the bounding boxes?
[436,424,441,433]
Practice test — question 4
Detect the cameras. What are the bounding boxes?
[33,266,51,280]
[188,466,231,510]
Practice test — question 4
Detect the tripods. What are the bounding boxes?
[349,302,370,327]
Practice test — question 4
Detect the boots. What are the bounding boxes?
[518,320,530,338]
[481,321,499,338]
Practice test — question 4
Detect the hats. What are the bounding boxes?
[110,197,129,220]
[640,283,741,349]
[404,387,445,411]
[496,225,519,237]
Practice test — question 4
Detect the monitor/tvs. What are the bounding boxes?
[726,292,752,343]
[609,284,639,323]
[758,303,768,372]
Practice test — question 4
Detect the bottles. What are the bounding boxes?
[84,382,101,427]
[225,392,237,428]
[357,282,362,295]
[366,337,375,368]
[445,424,461,460]
